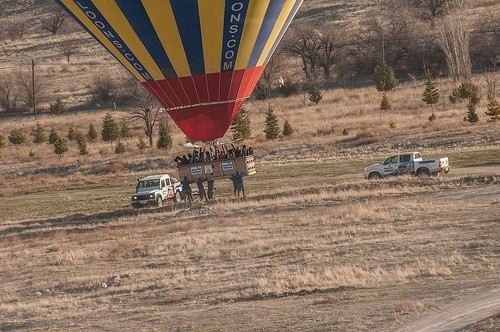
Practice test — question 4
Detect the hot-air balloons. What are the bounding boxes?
[55,0,303,146]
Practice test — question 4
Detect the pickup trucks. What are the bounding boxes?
[362,152,450,179]
[131,174,183,208]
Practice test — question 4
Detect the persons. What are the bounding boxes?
[173,140,253,167]
[180,171,246,204]
[278,75,284,87]
[135,178,139,193]
